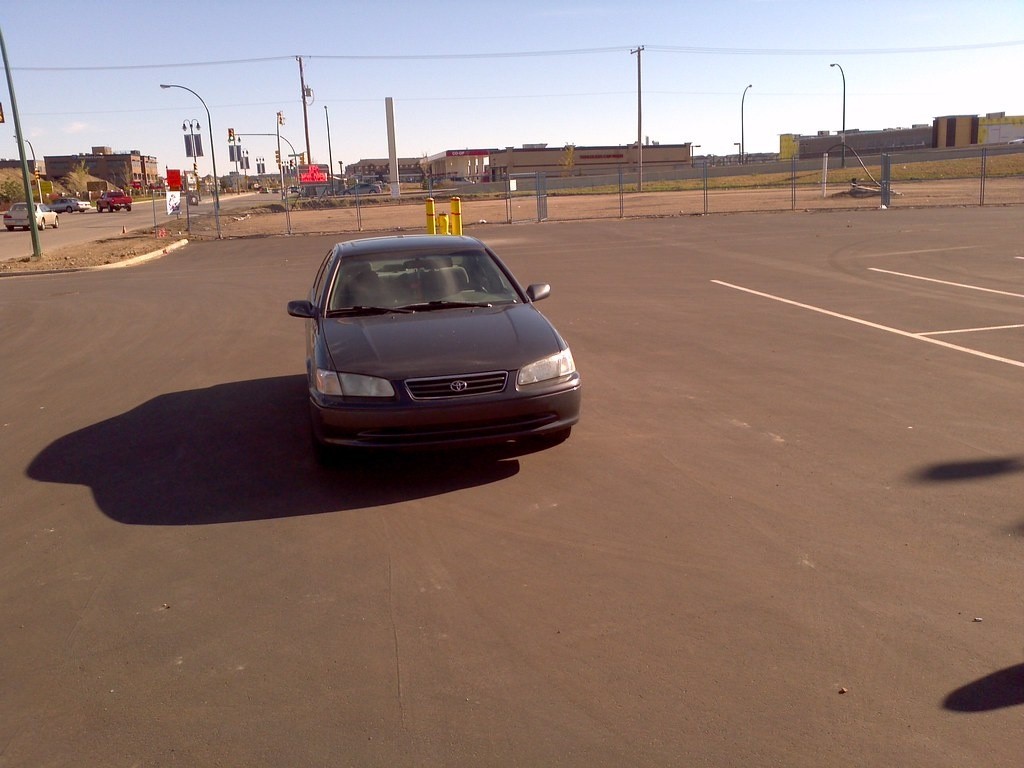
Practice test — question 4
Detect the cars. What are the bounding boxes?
[288,234,583,475]
[1009,138,1024,144]
[47,197,91,213]
[338,183,381,195]
[4,202,59,231]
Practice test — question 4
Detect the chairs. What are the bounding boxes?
[374,264,473,311]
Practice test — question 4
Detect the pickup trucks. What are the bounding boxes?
[97,192,132,212]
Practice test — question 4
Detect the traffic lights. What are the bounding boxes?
[280,111,286,124]
[300,152,304,164]
[290,160,294,170]
[275,150,281,163]
[228,128,235,141]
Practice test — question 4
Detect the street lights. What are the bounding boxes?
[830,63,846,169]
[324,105,334,196]
[160,84,220,211]
[228,134,242,195]
[240,147,249,192]
[183,119,202,202]
[742,85,753,165]
[14,136,43,203]
[256,156,265,190]
